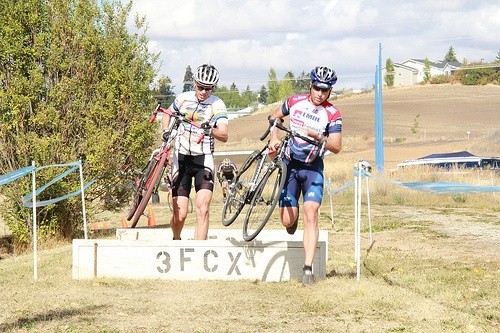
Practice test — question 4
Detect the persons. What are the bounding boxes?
[162,64,230,240]
[268,65,342,284]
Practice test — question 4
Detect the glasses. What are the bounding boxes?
[312,84,330,92]
[197,85,212,93]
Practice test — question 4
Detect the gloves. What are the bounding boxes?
[161,129,171,142]
[200,127,212,136]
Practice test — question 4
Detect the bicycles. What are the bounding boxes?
[126,102,207,228]
[221,114,329,243]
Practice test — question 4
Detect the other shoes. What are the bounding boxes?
[286,217,297,234]
[302,263,315,286]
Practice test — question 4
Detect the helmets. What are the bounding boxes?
[194,64,220,88]
[310,65,337,88]
